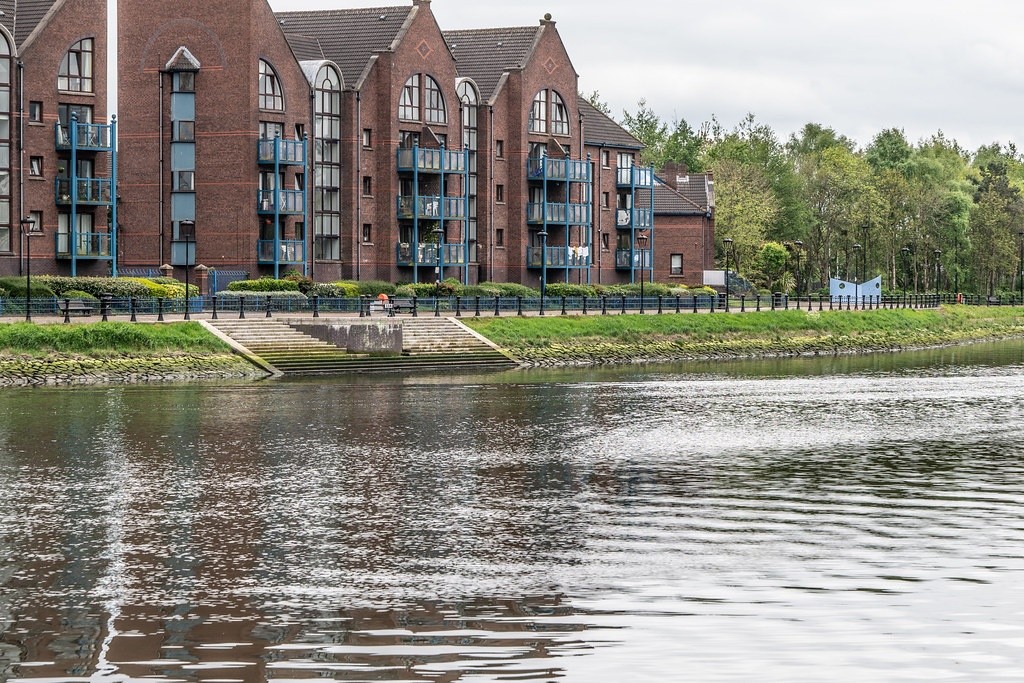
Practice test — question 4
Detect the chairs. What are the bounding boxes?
[426,201,439,216]
[281,245,294,259]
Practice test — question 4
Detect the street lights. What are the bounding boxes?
[637,233,648,313]
[853,243,862,310]
[723,237,733,312]
[537,229,549,315]
[934,248,942,307]
[432,227,445,317]
[794,239,803,309]
[20,215,36,323]
[902,246,910,307]
[181,217,195,320]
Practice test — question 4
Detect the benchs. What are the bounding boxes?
[57,301,94,317]
[985,297,1002,304]
[391,298,419,313]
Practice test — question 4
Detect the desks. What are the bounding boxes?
[63,132,71,145]
[281,197,286,211]
[82,131,95,145]
